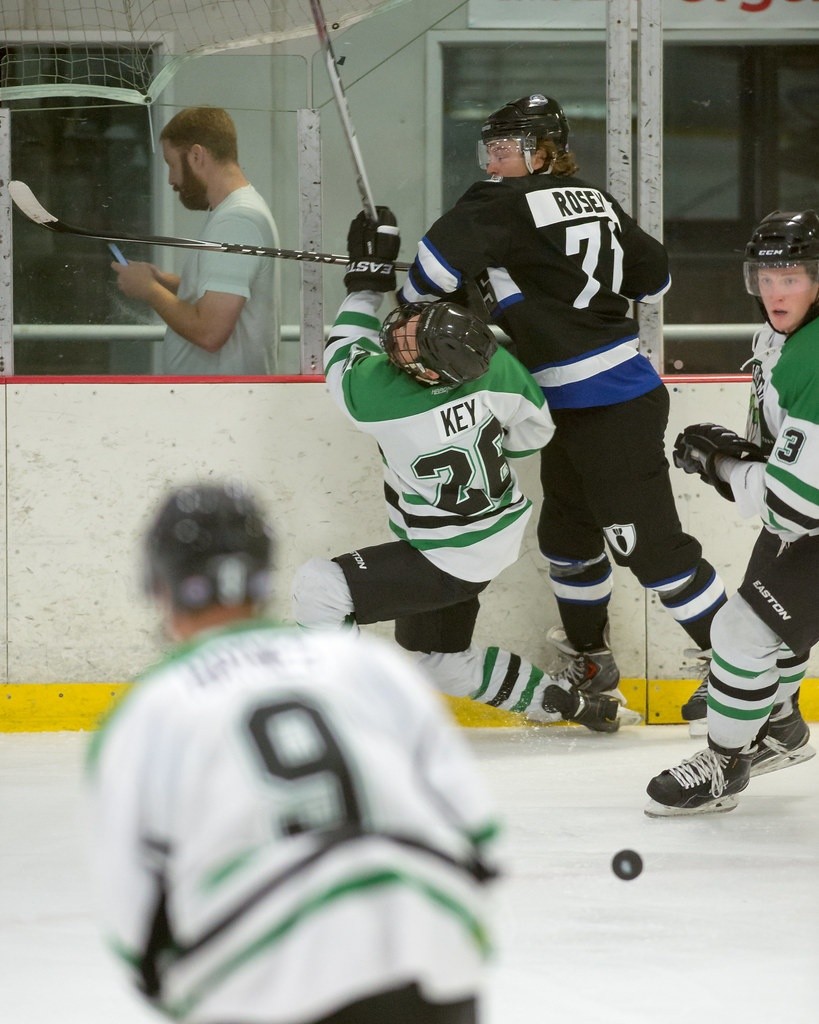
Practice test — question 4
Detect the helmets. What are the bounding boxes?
[378,300,499,389]
[480,94,570,145]
[743,209,819,261]
[140,484,270,611]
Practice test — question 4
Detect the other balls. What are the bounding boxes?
[611,849,645,882]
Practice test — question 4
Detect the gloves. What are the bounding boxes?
[342,206,401,292]
[672,421,768,502]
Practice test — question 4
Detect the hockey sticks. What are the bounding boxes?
[6,177,418,275]
[306,1,386,226]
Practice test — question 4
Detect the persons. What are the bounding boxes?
[287,206,643,735]
[643,209,819,819]
[84,486,496,1024]
[394,95,730,739]
[111,107,282,376]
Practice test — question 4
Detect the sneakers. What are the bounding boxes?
[641,715,770,816]
[544,617,628,707]
[681,647,713,736]
[541,683,644,736]
[749,686,817,778]
